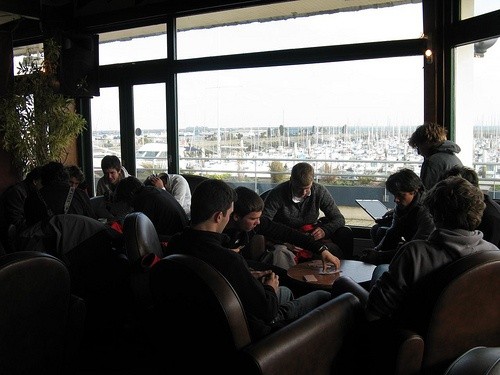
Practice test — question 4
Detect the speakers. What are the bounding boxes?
[58,31,100,98]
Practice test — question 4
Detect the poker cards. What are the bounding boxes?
[319,263,342,274]
[304,274,317,282]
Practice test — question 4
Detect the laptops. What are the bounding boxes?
[355,199,390,220]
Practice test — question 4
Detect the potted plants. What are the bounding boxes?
[0,39,90,215]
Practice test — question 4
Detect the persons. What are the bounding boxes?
[410,123,460,187]
[223,186,341,271]
[167,179,294,318]
[365,169,436,263]
[333,176,499,304]
[0,156,194,256]
[262,163,345,256]
[437,165,500,249]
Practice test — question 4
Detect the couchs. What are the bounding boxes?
[123,212,164,267]
[149,254,362,375]
[0,251,86,375]
[333,250,500,375]
[182,174,209,195]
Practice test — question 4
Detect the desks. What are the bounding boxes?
[317,206,377,240]
[287,260,377,286]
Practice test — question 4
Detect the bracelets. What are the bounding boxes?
[319,246,328,254]
